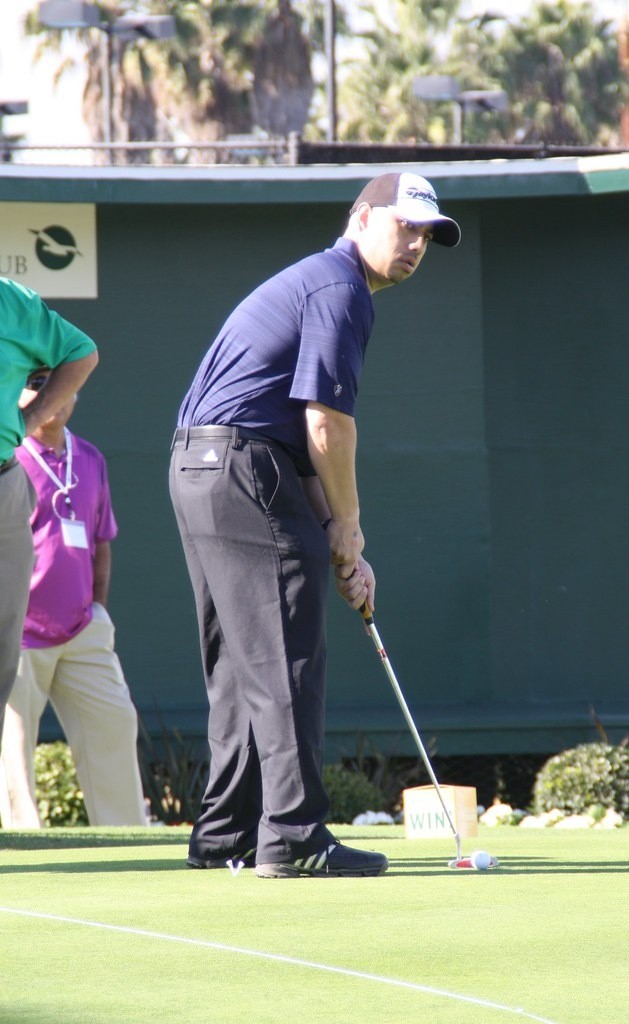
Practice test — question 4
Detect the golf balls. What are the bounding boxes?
[470,850,491,870]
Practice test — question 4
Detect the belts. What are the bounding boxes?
[176,425,278,443]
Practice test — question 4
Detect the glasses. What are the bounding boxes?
[25,375,49,391]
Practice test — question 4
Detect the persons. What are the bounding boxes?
[0,280,101,743]
[168,175,461,880]
[0,363,154,832]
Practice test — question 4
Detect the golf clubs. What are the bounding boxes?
[320,516,500,869]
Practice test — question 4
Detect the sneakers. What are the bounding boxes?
[186,851,255,871]
[255,838,389,879]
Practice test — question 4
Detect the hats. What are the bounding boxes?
[350,171,462,248]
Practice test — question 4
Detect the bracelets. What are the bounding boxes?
[323,519,333,531]
